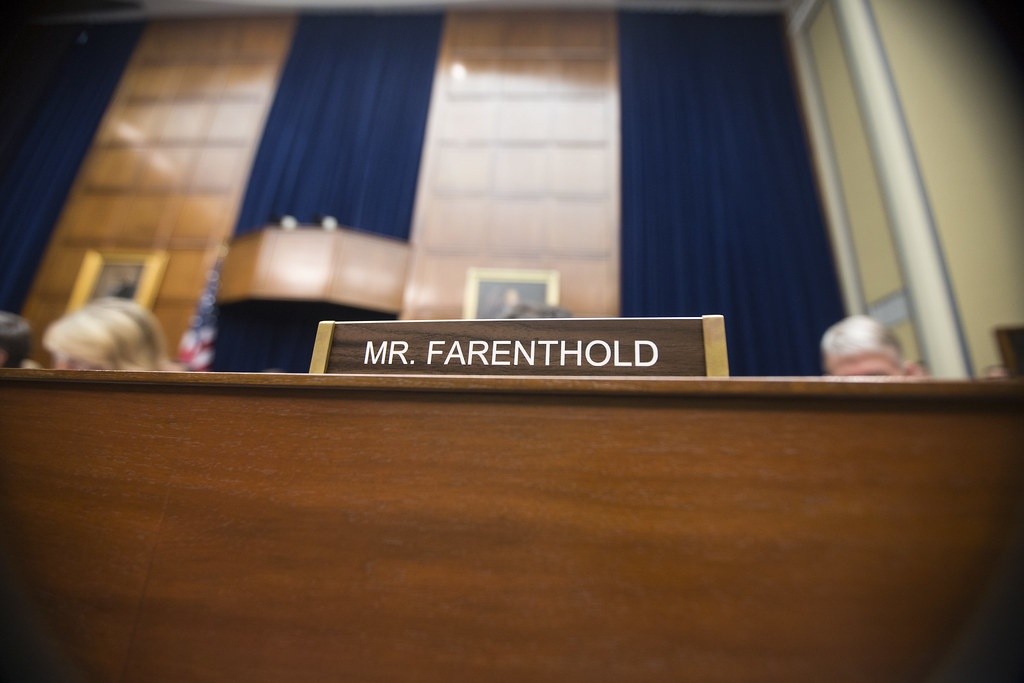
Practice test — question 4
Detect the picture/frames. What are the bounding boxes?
[463,267,560,321]
[64,247,171,319]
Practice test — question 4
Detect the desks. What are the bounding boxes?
[0,368,1024,682]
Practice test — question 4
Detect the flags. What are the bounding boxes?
[175,258,226,370]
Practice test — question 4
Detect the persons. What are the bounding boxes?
[43,295,174,370]
[0,309,41,368]
[821,311,902,377]
